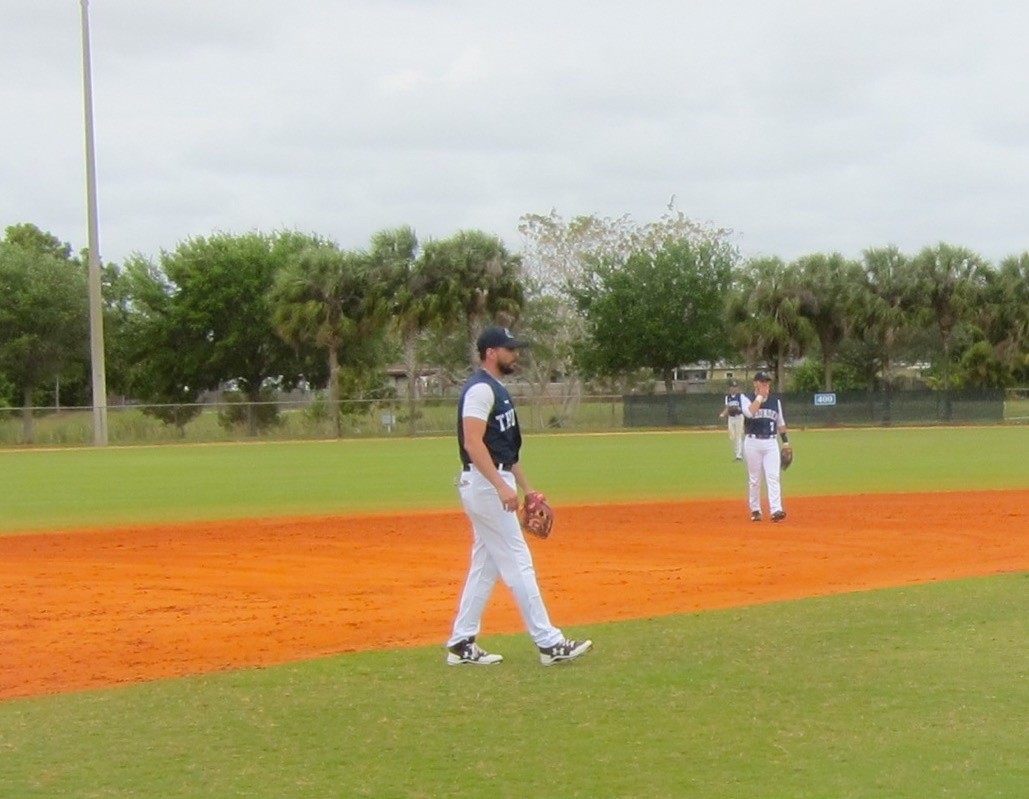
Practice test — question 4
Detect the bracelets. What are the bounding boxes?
[755,394,763,403]
[783,442,789,446]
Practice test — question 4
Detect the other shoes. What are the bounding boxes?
[736,457,742,461]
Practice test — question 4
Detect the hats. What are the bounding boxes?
[476,326,529,348]
[729,381,737,386]
[753,372,772,382]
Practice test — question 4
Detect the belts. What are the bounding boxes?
[749,434,776,439]
[463,463,512,471]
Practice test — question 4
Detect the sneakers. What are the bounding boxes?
[539,638,592,666]
[751,510,762,521]
[772,511,786,521]
[447,634,505,664]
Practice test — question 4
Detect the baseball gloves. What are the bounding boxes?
[780,446,793,471]
[521,490,555,541]
[728,405,738,417]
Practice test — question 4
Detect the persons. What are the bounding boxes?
[740,372,793,523]
[720,382,744,460]
[446,326,594,667]
